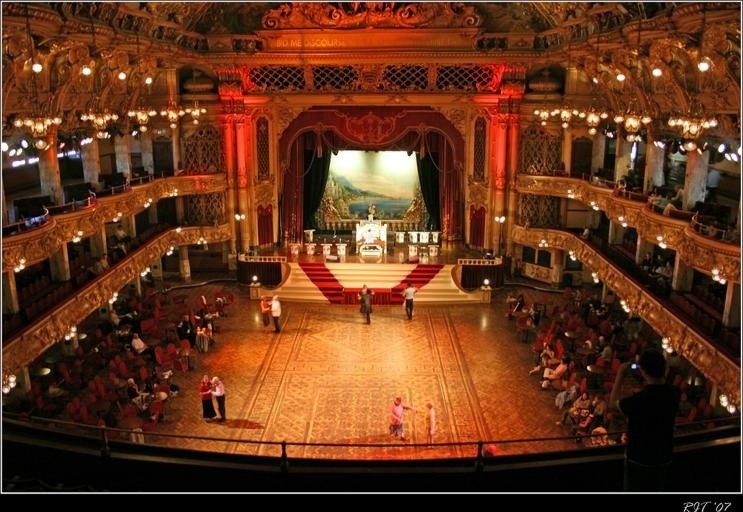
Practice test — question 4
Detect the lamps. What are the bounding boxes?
[12,6,62,153]
[612,4,653,146]
[125,20,159,130]
[185,36,207,126]
[551,7,578,128]
[161,28,186,130]
[576,12,610,138]
[668,8,722,152]
[533,47,558,129]
[80,6,119,143]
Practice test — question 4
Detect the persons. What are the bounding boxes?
[401,282,417,319]
[425,404,436,448]
[389,396,412,440]
[608,347,682,491]
[358,284,374,325]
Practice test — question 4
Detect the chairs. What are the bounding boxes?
[502,184,742,445]
[1,168,231,440]
